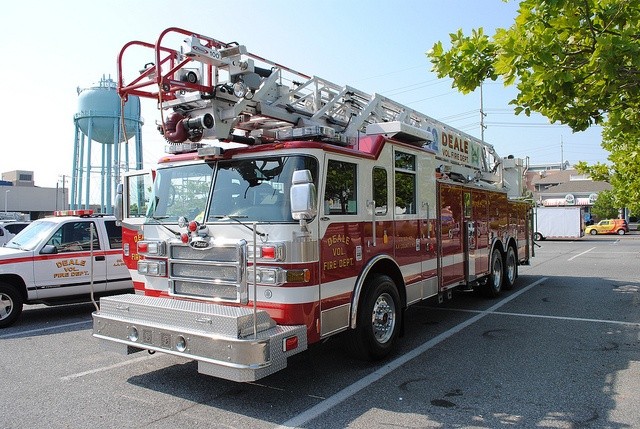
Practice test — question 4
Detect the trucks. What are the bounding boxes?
[533,205,587,241]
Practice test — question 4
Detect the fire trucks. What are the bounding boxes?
[90,26,541,384]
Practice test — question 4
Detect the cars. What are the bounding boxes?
[0,209,135,330]
[0,220,35,247]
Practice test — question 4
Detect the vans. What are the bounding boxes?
[585,218,630,235]
